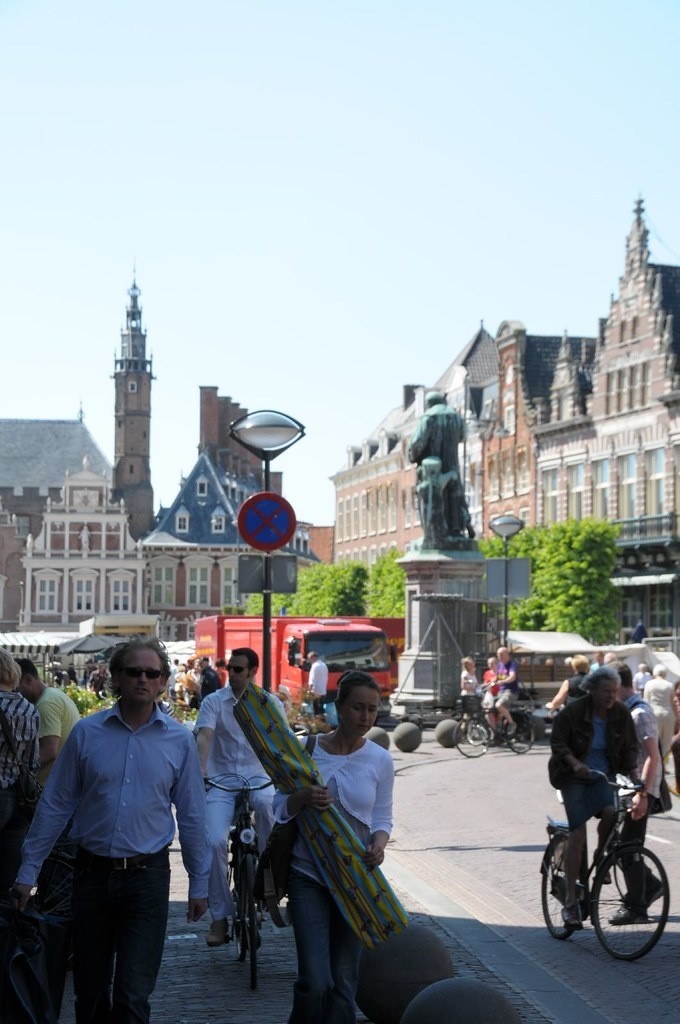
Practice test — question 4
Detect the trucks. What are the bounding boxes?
[192,614,394,729]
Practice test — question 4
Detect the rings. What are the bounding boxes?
[319,794,322,799]
[320,788,322,793]
[378,852,382,856]
[640,812,645,816]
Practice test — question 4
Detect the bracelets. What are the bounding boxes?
[635,790,648,798]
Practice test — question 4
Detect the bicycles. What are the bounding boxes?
[452,679,536,758]
[200,772,277,992]
[538,768,672,962]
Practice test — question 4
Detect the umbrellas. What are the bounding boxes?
[58,632,119,663]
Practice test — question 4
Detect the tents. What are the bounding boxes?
[498,629,596,691]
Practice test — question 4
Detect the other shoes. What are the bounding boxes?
[608,906,649,924]
[507,723,516,737]
[623,876,664,911]
[562,907,583,930]
[206,916,230,946]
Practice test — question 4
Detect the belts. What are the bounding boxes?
[82,856,168,871]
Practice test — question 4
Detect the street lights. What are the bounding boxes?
[488,514,524,647]
[226,408,307,695]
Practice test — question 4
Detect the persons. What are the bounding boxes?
[194,646,296,947]
[630,619,648,643]
[544,655,594,707]
[54,657,112,702]
[270,667,394,1024]
[167,655,227,713]
[479,646,520,743]
[0,647,82,901]
[461,656,482,741]
[10,636,221,1024]
[548,666,647,928]
[605,658,663,925]
[307,648,329,717]
[589,651,680,794]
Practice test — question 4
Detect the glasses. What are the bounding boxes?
[118,666,164,681]
[227,664,253,674]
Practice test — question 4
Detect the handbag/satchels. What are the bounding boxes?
[253,820,296,909]
[648,779,672,814]
[17,770,43,816]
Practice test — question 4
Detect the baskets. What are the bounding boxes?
[456,694,480,713]
